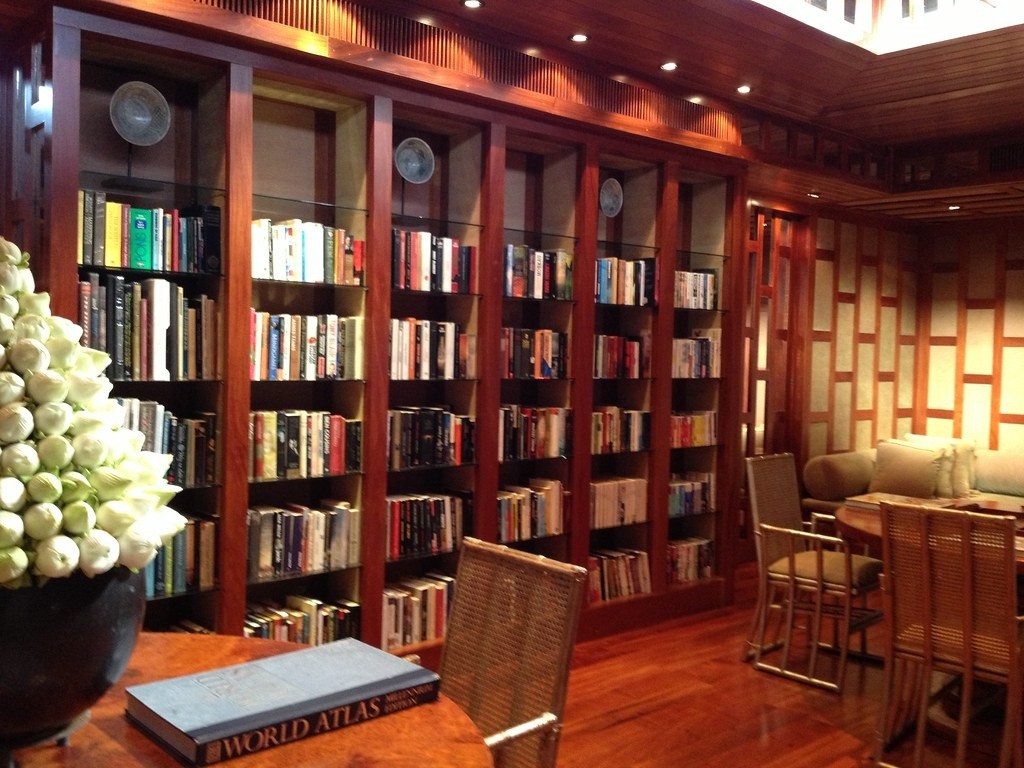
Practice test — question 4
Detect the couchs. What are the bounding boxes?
[803,448,1024,513]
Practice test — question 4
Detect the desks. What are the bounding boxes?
[805,492,1024,666]
[13,633,497,767]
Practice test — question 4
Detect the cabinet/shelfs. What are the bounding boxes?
[11,5,747,658]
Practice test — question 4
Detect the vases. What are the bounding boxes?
[0,567,145,754]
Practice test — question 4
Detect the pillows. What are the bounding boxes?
[901,436,956,497]
[952,442,969,497]
[867,437,943,499]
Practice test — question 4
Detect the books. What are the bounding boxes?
[78,184,721,647]
[125,637,441,767]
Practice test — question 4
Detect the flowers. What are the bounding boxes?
[0,236,186,588]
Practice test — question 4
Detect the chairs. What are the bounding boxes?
[870,500,1023,768]
[742,454,881,694]
[427,537,584,768]
